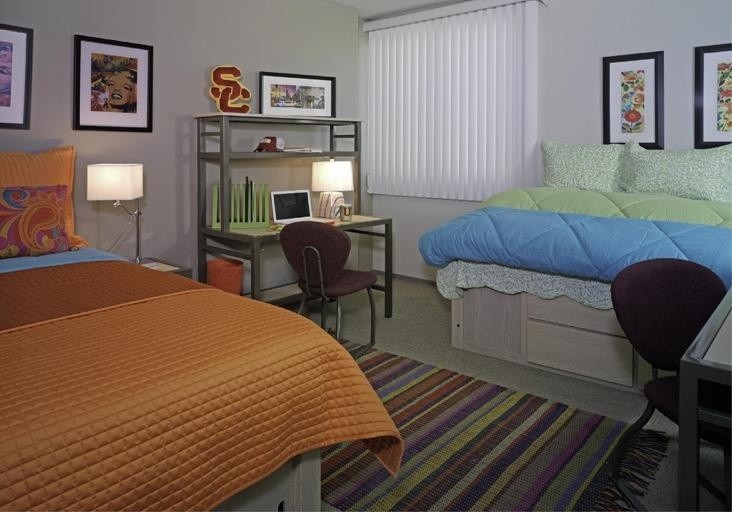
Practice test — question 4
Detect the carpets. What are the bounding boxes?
[317,326,670,512]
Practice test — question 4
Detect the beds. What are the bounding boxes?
[0,143,403,512]
[420,135,732,397]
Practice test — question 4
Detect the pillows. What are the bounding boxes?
[540,135,623,192]
[0,185,80,260]
[615,138,730,203]
[0,145,90,250]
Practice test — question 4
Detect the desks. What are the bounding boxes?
[196,214,396,321]
[676,291,732,512]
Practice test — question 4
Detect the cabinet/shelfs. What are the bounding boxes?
[193,115,362,229]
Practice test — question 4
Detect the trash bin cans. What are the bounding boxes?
[207,259,243,295]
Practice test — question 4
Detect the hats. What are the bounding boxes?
[254,136,284,153]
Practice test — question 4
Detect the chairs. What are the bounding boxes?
[281,220,377,346]
[613,258,732,512]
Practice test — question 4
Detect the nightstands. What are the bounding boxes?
[132,252,191,278]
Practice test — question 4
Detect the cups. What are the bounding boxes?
[339,204,353,222]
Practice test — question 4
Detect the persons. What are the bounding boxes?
[91,64,137,112]
[0,47,11,105]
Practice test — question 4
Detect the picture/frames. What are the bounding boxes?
[600,47,667,151]
[69,33,154,134]
[0,21,35,130]
[692,43,730,150]
[258,68,338,117]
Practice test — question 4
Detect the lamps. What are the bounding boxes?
[86,162,146,263]
[309,158,356,217]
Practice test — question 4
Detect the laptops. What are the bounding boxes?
[270,190,335,226]
